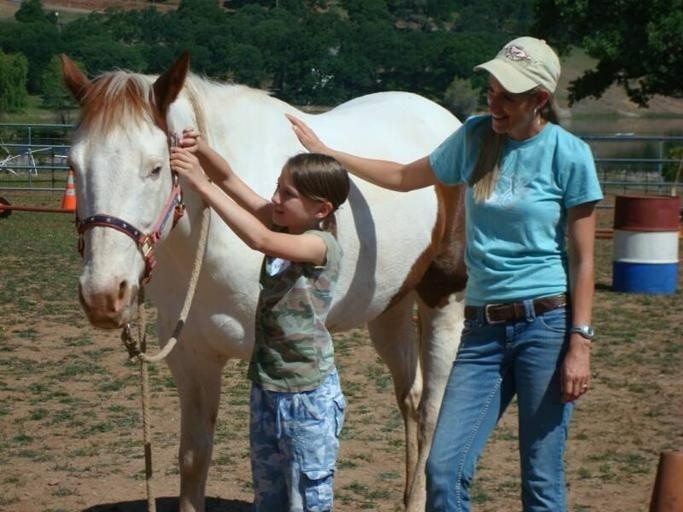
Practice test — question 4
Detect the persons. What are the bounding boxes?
[168,126,352,512]
[283,35,605,512]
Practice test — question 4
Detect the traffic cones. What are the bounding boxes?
[58,168,78,211]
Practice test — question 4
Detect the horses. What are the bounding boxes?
[59,52,465,512]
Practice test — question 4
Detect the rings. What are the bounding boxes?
[578,383,587,389]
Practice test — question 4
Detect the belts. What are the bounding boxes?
[464,294,569,325]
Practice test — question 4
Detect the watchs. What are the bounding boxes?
[567,326,594,339]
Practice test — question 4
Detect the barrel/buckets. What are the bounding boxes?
[612,194,679,294]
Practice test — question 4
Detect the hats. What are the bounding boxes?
[474,36,562,95]
